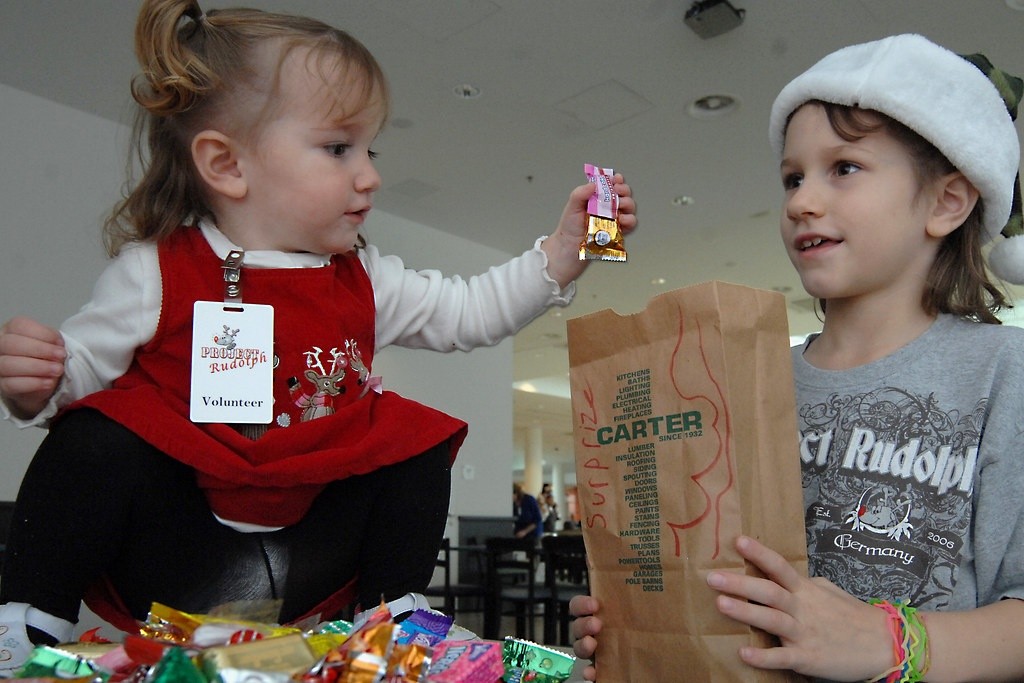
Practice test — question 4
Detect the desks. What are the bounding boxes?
[450,545,586,639]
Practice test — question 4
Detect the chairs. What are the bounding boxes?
[426,536,591,647]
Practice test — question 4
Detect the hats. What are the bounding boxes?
[768,32,1024,287]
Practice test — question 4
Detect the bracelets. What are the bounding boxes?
[868,597,930,683]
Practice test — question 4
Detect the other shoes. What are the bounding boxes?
[354,595,483,649]
[2,599,74,680]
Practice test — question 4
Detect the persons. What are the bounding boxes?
[570,33,1024,683]
[0,0,637,679]
[514,482,543,573]
[538,483,578,532]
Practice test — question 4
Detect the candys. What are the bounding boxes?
[578,162,627,262]
[0,596,578,683]
